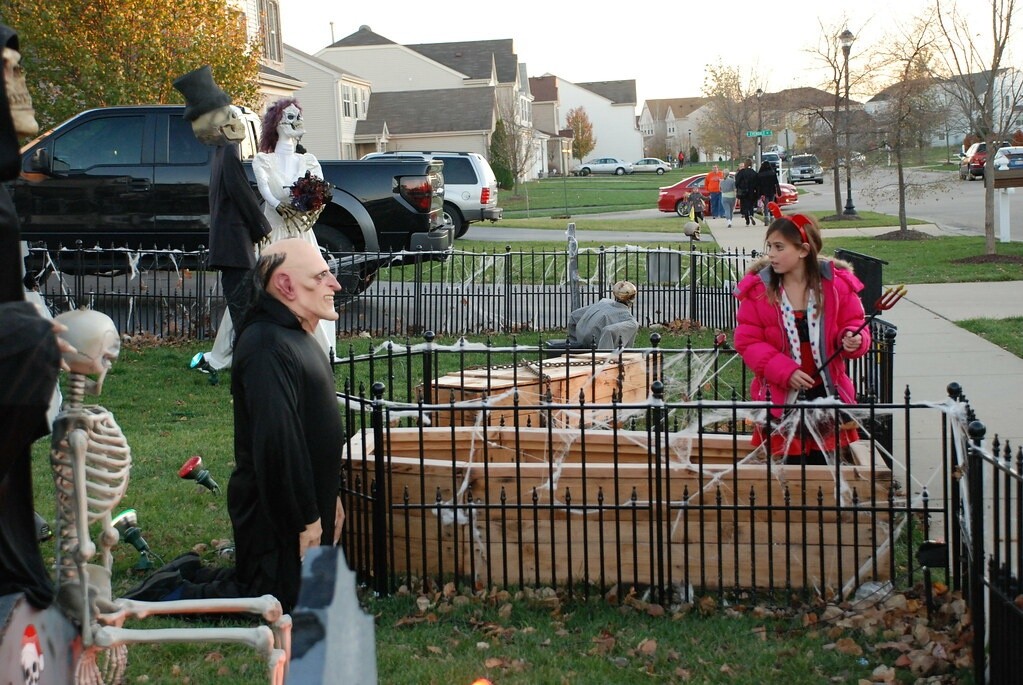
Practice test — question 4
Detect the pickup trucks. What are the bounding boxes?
[4,105,456,309]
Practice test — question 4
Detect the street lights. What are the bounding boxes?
[754,89,763,172]
[839,24,856,215]
[688,129,693,168]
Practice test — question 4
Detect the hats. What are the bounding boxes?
[172,64,233,120]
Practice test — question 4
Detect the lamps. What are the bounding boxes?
[109,508,156,570]
[178,454,222,497]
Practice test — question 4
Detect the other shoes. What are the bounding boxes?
[697,214,775,228]
[143,551,203,580]
[121,564,183,601]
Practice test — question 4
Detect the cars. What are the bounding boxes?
[569,157,633,175]
[625,157,672,175]
[983,147,1023,180]
[751,153,782,176]
[959,140,1012,182]
[658,171,799,217]
[786,154,824,185]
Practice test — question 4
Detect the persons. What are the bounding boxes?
[735,202,871,466]
[754,161,782,227]
[678,151,685,169]
[666,152,673,164]
[720,170,738,229]
[705,165,727,220]
[734,160,760,226]
[687,186,712,225]
[115,239,347,623]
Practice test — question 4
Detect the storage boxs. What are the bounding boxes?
[425,348,660,428]
[333,425,891,590]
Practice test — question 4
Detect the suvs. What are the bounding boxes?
[357,152,503,240]
